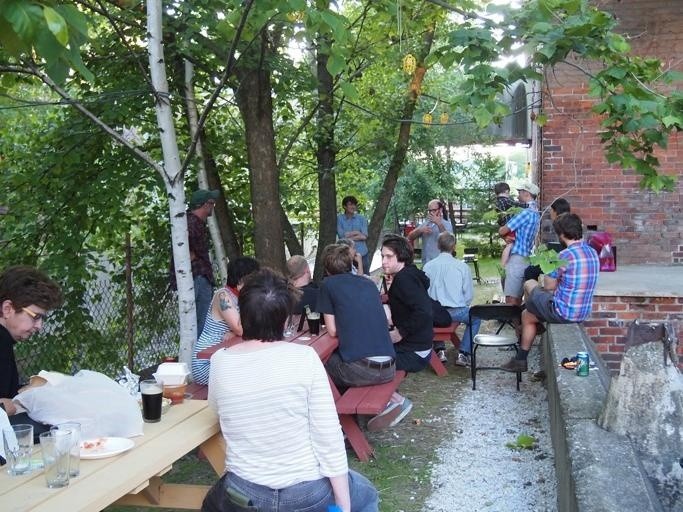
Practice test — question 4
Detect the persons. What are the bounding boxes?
[409,199,453,267]
[190,257,260,388]
[199,267,379,512]
[336,195,369,275]
[0,265,62,465]
[314,244,396,388]
[494,182,532,278]
[499,212,600,372]
[187,188,220,340]
[421,231,482,368]
[285,255,317,314]
[337,238,380,286]
[366,234,434,433]
[498,181,541,324]
[516,198,570,337]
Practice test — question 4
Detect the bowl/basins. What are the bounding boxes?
[140,398,172,416]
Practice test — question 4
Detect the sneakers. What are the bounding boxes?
[455,353,473,367]
[386,399,413,427]
[434,349,449,362]
[501,357,528,373]
[367,403,402,433]
[517,323,546,335]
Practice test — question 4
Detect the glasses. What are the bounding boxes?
[426,208,440,212]
[22,307,43,321]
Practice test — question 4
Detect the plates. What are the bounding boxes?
[70,436,135,459]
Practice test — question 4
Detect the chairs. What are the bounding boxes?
[469,304,524,390]
[463,247,480,282]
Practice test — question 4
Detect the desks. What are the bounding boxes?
[0,399,221,512]
[197,313,338,365]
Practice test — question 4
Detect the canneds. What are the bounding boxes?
[576,352,589,376]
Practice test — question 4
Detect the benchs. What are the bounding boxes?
[336,368,406,415]
[428,319,459,377]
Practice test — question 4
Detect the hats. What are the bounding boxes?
[189,190,221,206]
[516,182,541,196]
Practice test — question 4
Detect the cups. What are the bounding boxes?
[307,313,320,335]
[140,380,163,423]
[3,424,34,475]
[40,431,72,489]
[50,422,82,477]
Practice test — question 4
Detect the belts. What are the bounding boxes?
[358,357,396,369]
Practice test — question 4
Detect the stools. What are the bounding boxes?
[186,381,210,459]
